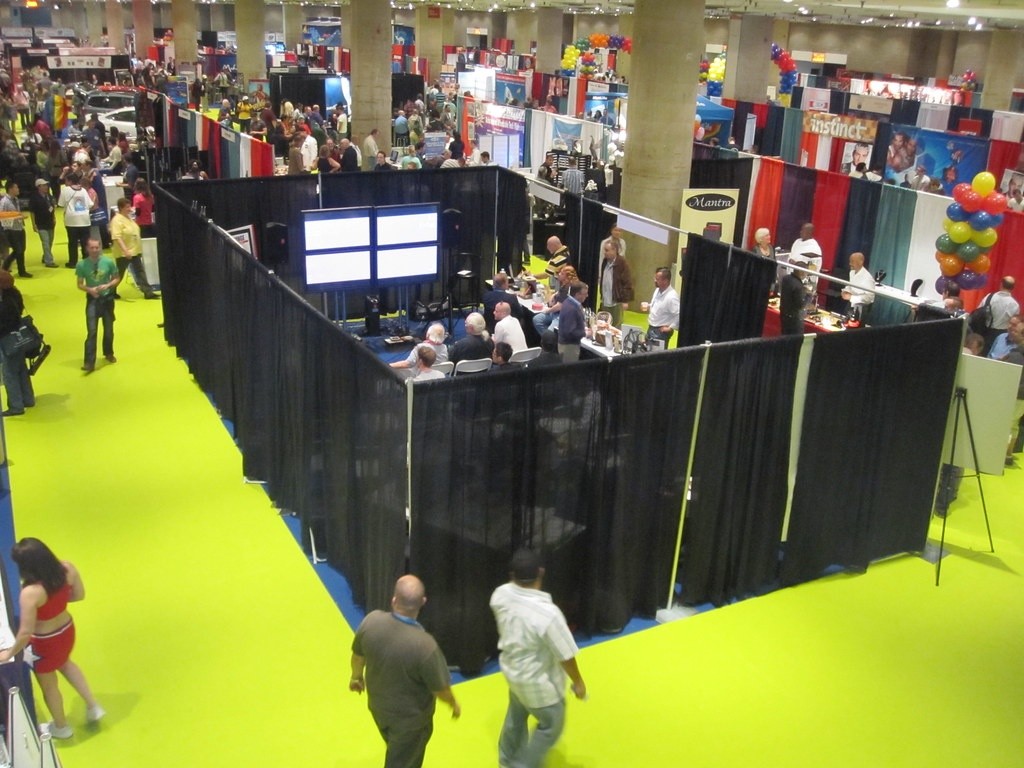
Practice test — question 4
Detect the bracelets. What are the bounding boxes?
[351,677,363,683]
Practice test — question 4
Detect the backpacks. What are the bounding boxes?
[969,294,993,334]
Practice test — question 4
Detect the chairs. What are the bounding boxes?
[816,266,851,312]
[396,367,416,381]
[455,358,492,376]
[509,347,541,363]
[417,361,454,378]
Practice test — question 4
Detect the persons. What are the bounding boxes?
[1002,172,1024,200]
[388,222,679,382]
[489,548,586,768]
[841,132,918,173]
[0,171,159,418]
[508,94,614,264]
[0,52,208,220]
[393,46,499,169]
[709,222,884,335]
[933,189,1024,518]
[349,574,461,768]
[202,49,392,175]
[694,136,759,161]
[595,67,627,84]
[849,162,945,196]
[0,537,106,739]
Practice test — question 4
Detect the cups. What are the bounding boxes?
[641,302,648,313]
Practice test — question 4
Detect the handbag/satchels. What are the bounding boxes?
[0,325,36,357]
[90,207,108,226]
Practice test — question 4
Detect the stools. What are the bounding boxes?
[453,270,480,314]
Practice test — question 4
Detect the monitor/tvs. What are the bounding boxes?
[301,202,442,294]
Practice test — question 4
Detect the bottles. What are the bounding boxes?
[500,268,506,274]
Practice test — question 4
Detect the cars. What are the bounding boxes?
[85,105,139,141]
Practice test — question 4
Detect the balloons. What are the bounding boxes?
[561,33,631,78]
[693,114,705,141]
[935,172,1008,295]
[699,42,798,107]
[163,30,172,46]
[960,68,976,93]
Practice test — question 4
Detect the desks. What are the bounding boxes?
[486,274,563,314]
[554,311,646,357]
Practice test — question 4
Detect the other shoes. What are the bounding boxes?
[106,354,116,362]
[81,365,94,371]
[145,294,161,299]
[46,262,59,268]
[2,410,24,416]
[24,403,35,407]
[20,271,33,278]
[65,263,76,268]
[112,294,120,298]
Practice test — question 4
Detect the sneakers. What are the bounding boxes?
[39,721,73,739]
[86,706,104,722]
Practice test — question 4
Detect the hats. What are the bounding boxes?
[512,546,549,579]
[0,268,14,289]
[35,179,49,187]
[68,141,82,148]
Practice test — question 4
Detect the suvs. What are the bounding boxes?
[66,81,136,119]
